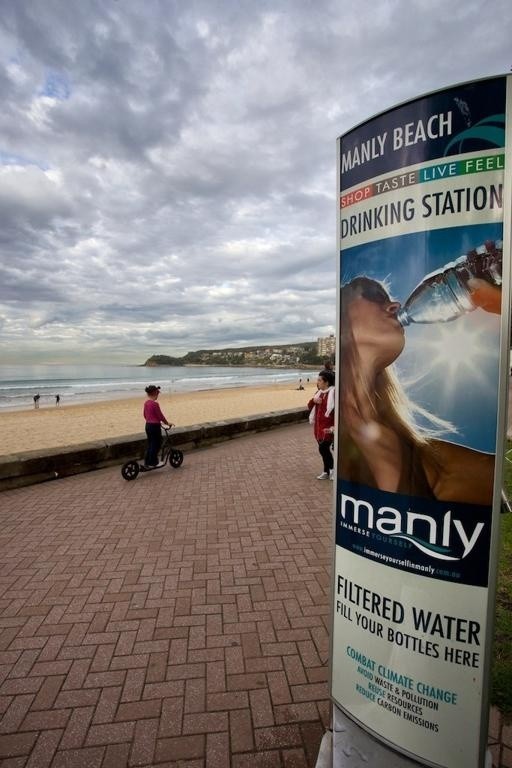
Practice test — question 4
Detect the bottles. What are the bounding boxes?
[396,243,503,326]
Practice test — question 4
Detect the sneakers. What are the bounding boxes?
[316,467,335,480]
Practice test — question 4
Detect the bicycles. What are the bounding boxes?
[121,424,183,480]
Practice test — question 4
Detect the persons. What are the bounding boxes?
[308,358,335,481]
[34,394,40,408]
[144,385,172,468]
[55,395,59,407]
[337,241,502,506]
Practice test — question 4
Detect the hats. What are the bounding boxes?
[143,383,162,392]
